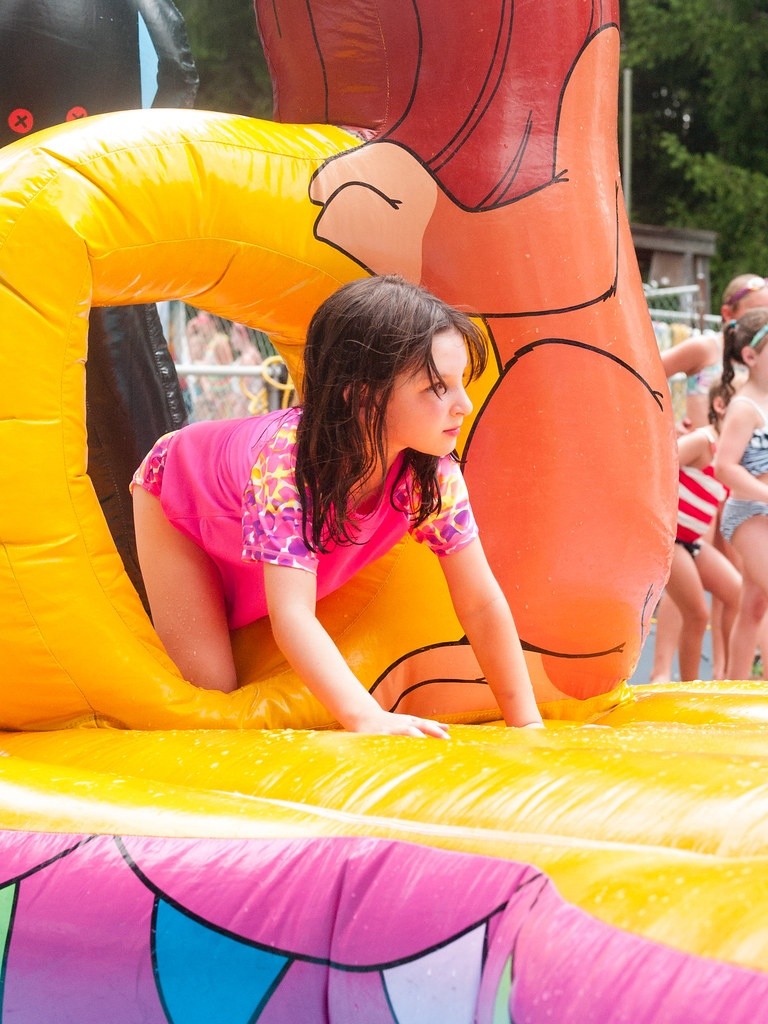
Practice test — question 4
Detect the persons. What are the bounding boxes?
[184,312,266,415]
[128,274,547,739]
[650,273,768,683]
